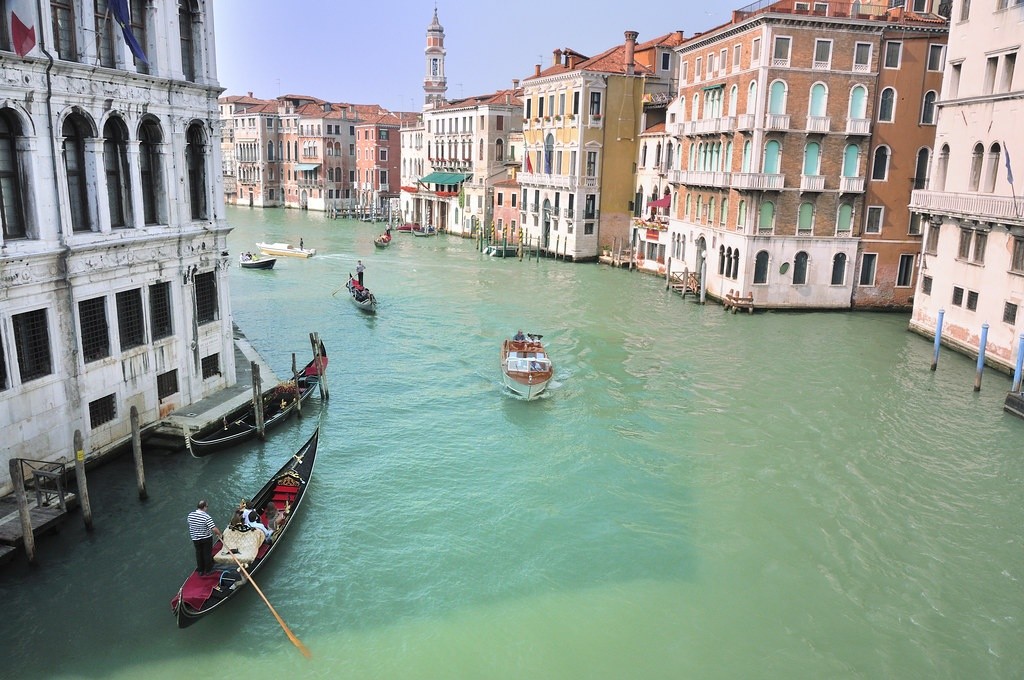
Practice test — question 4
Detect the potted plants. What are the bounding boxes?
[602,245,609,256]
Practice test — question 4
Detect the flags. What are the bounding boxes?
[105,0,149,65]
[1005,149,1014,184]
[10,11,36,58]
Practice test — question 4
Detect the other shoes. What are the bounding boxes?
[198,572,204,576]
[206,570,215,576]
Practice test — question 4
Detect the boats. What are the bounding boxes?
[240,258,277,270]
[500,331,555,401]
[373,233,392,248]
[174,425,319,639]
[189,339,328,462]
[396,222,421,233]
[348,273,378,314]
[256,242,317,259]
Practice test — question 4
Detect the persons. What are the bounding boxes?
[231,502,284,543]
[357,260,371,302]
[239,251,260,262]
[374,222,392,243]
[187,500,223,576]
[513,330,526,343]
[300,238,303,251]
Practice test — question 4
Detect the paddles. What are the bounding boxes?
[332,268,364,296]
[216,535,313,661]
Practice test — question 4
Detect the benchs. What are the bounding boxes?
[213,523,266,565]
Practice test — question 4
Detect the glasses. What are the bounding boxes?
[205,505,209,508]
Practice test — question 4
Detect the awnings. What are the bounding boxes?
[647,194,672,207]
[417,171,474,185]
[294,163,322,170]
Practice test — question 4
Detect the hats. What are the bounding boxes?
[244,502,254,509]
